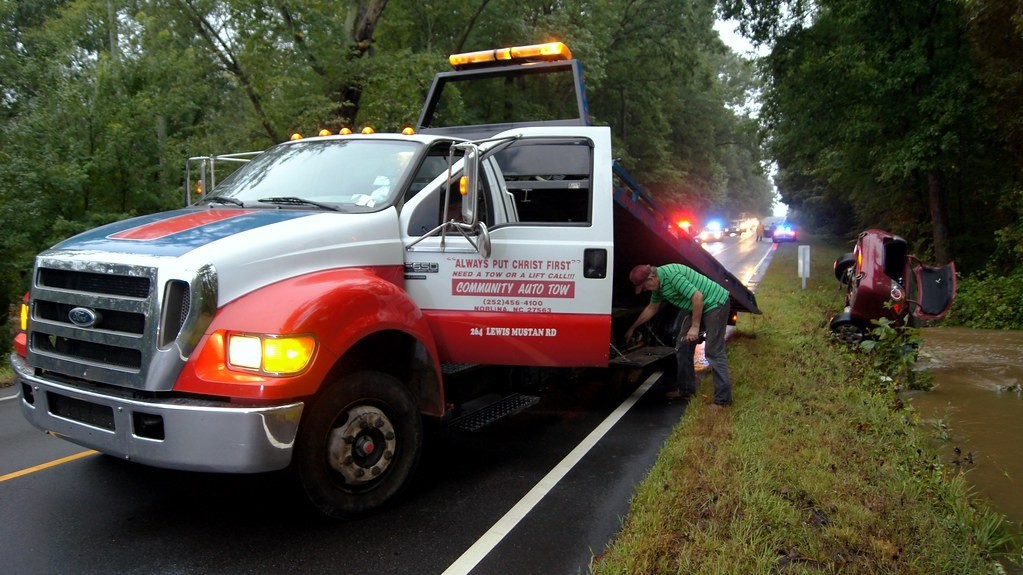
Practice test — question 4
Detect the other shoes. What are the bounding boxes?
[666,391,695,400]
[708,402,731,411]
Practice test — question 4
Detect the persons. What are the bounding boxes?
[625,262,733,410]
[756,221,764,242]
[769,222,776,230]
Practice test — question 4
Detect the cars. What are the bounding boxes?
[829,228,956,360]
[763,216,797,243]
[699,213,758,242]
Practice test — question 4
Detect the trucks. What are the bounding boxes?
[5,41,761,523]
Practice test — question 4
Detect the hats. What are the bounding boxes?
[629,264,651,294]
